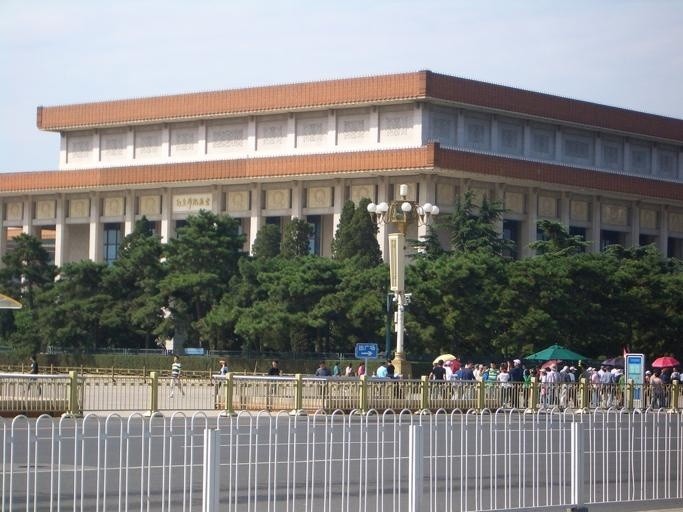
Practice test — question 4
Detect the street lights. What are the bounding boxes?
[366,182,441,378]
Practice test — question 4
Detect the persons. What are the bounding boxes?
[375,362,387,377]
[332,361,342,375]
[267,360,280,394]
[386,359,395,378]
[212,359,228,396]
[314,361,330,399]
[428,357,683,412]
[344,362,353,376]
[167,353,186,400]
[22,355,42,396]
[357,362,364,377]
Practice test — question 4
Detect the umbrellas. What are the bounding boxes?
[520,342,591,371]
[431,354,456,366]
